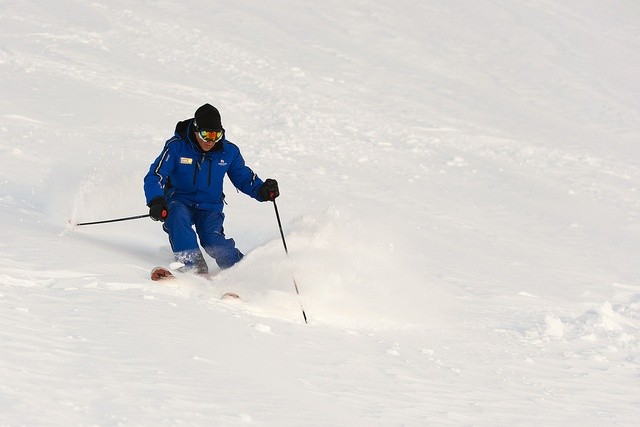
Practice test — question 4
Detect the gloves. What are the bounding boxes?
[260,179,279,201]
[148,197,167,221]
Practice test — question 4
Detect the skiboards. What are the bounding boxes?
[150,267,241,299]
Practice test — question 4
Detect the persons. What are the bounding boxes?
[144,103,280,275]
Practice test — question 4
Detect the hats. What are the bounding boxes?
[195,104,221,131]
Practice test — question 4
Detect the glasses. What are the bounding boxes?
[199,131,222,142]
